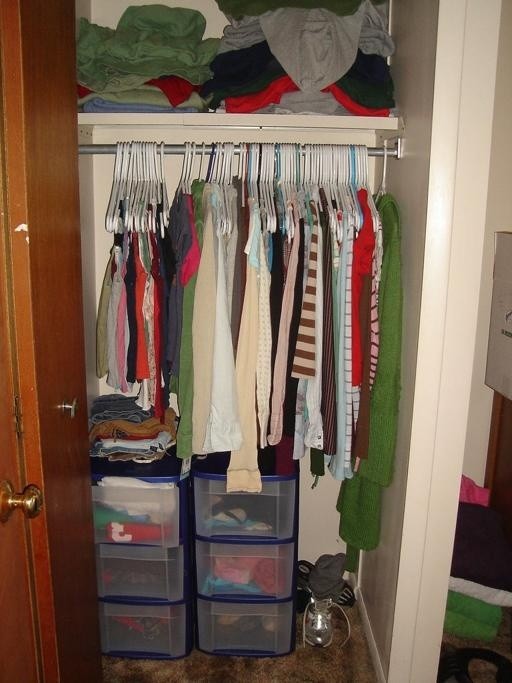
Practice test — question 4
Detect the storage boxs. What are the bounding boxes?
[91,472,300,659]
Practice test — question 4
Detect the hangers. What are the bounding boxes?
[105,140,396,245]
